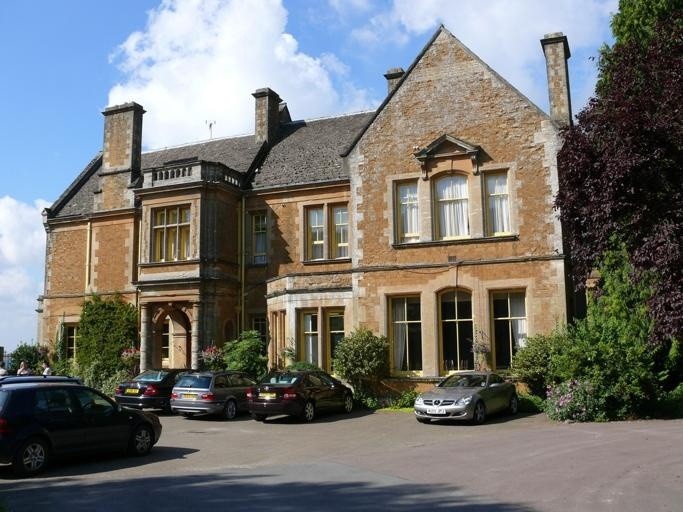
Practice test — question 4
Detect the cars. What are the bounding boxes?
[414,369,519,425]
[1,383,164,476]
[115,369,194,414]
[246,372,356,421]
[172,373,248,420]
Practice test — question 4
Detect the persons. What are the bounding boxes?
[17,362,30,376]
[0,360,8,376]
[42,361,52,376]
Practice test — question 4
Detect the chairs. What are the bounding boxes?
[53,393,65,406]
[290,377,297,384]
[270,377,277,385]
